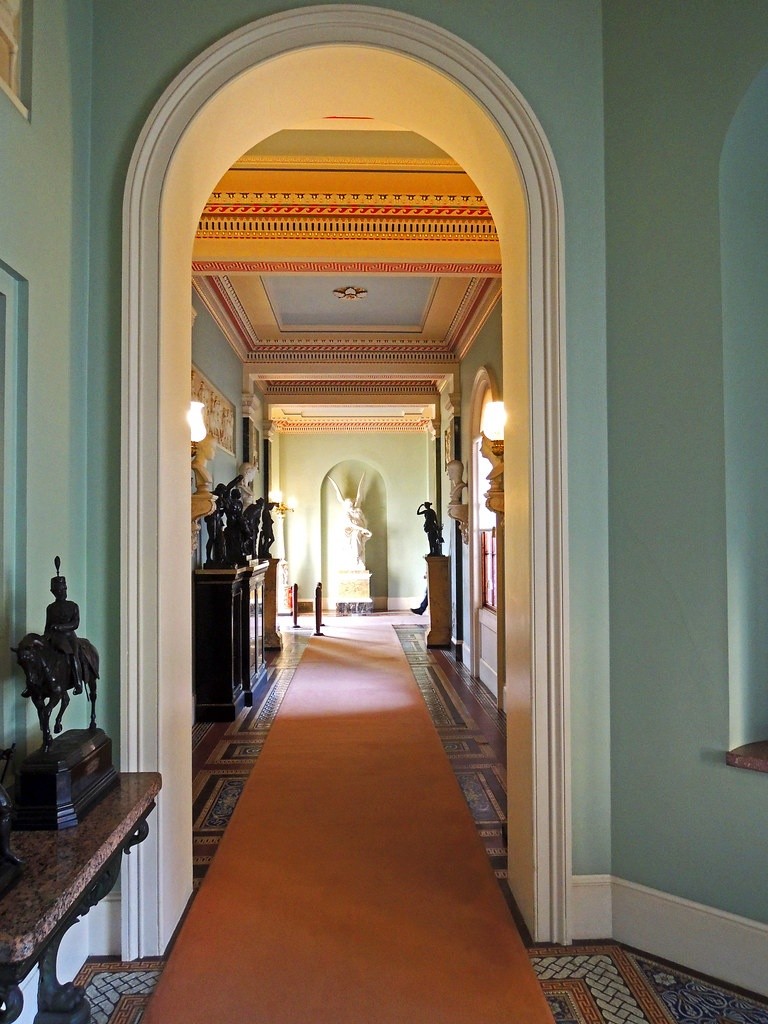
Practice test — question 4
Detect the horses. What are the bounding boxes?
[12,633,100,750]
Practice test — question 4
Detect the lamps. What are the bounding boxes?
[332,287,367,301]
[481,401,505,455]
[190,401,207,457]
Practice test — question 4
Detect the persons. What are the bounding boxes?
[190,432,217,493]
[478,428,503,492]
[445,459,469,506]
[20,555,83,699]
[202,462,275,571]
[410,588,428,616]
[417,501,445,558]
